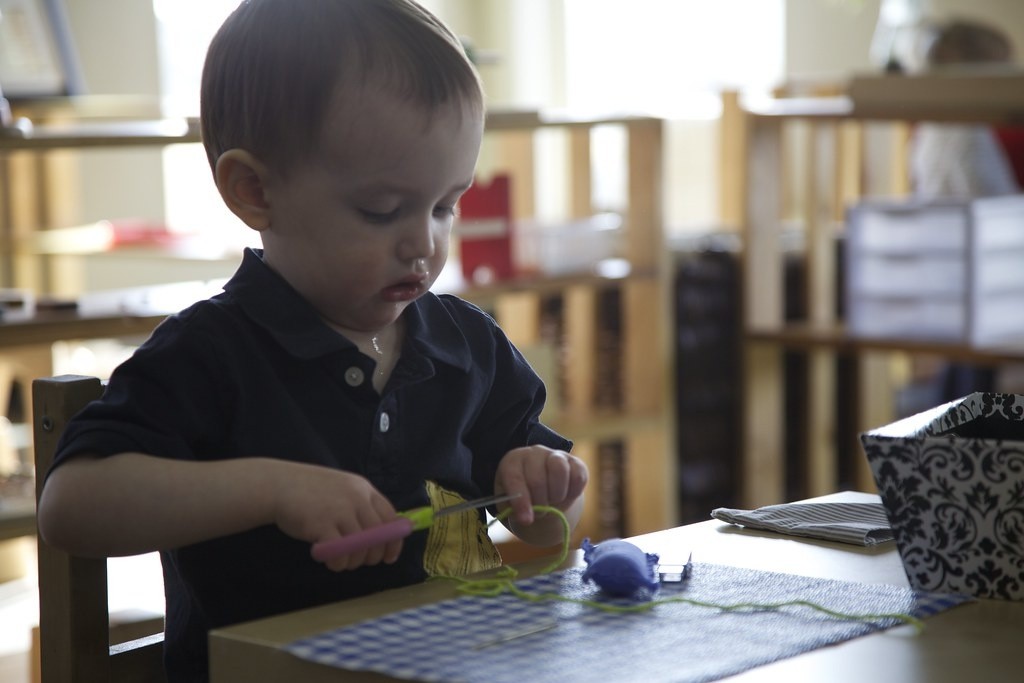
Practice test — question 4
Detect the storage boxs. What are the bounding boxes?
[853,191,1024,347]
[861,391,1024,602]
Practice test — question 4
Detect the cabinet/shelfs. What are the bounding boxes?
[0,63,1024,542]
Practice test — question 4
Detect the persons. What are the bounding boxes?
[38,1,588,683]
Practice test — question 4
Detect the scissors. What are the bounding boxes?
[310,491,523,565]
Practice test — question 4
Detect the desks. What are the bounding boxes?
[205,489,1024,683]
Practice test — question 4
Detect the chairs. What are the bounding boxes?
[30,372,166,683]
